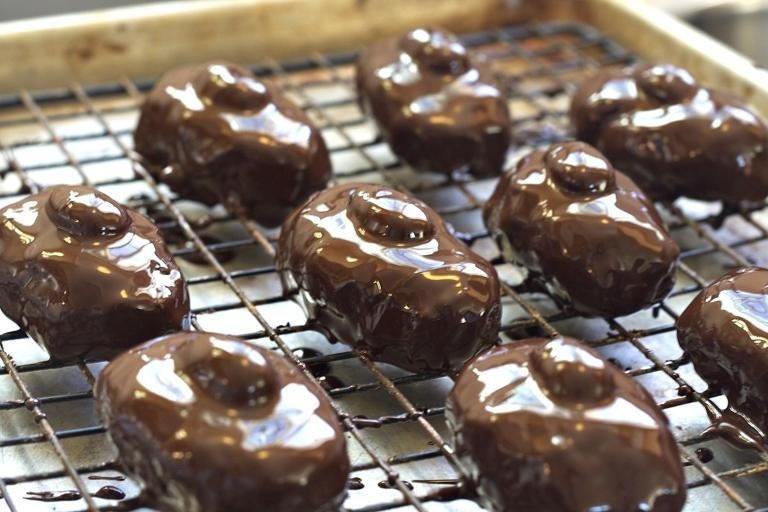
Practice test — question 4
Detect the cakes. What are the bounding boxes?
[276,185,502,375]
[355,24,510,181]
[443,336,686,512]
[132,58,332,228]
[0,188,190,363]
[93,333,352,512]
[572,63,768,204]
[676,267,768,448]
[482,142,680,317]
